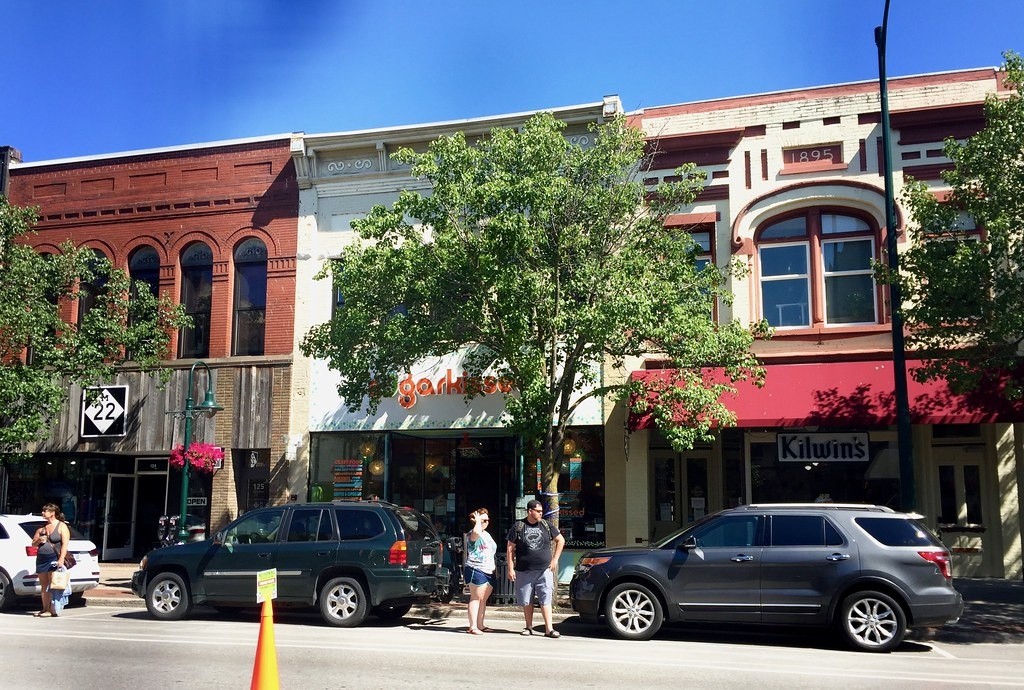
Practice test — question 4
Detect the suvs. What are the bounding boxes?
[130,499,452,627]
[568,502,965,651]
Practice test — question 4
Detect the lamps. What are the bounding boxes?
[564,432,576,455]
[358,437,385,475]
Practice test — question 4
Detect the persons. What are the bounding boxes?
[32,503,70,618]
[506,500,565,638]
[464,508,497,636]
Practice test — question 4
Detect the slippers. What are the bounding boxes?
[40,609,57,617]
[521,627,532,636]
[34,611,46,617]
[481,627,494,632]
[466,629,483,634]
[545,630,560,638]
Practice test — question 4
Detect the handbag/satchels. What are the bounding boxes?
[52,542,77,569]
[50,568,67,590]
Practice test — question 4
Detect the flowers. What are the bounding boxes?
[169,441,225,472]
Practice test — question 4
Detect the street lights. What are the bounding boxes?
[176,359,226,547]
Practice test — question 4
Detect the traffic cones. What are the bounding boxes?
[248,595,279,690]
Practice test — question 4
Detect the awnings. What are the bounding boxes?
[627,359,1024,430]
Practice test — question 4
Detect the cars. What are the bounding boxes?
[0,512,101,613]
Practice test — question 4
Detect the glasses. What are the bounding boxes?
[480,518,490,523]
[532,508,543,513]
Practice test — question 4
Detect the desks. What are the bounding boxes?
[776,303,808,325]
